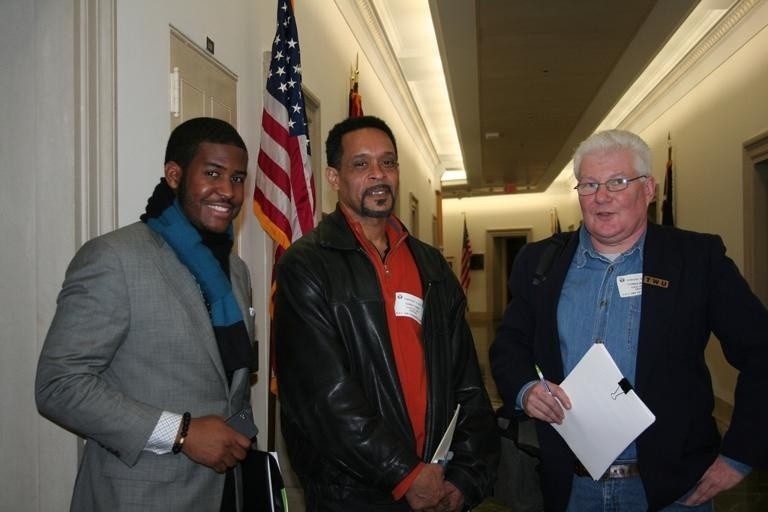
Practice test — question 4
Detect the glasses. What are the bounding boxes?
[570,174,650,197]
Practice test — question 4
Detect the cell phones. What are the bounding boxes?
[224,408,258,440]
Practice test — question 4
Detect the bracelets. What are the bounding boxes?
[170,411,192,457]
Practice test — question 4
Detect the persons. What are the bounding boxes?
[33,116,268,510]
[272,113,505,511]
[486,128,765,510]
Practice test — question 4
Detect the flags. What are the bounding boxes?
[248,2,322,396]
[656,161,677,224]
[459,221,474,291]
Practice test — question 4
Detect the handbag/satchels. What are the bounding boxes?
[241,449,285,512]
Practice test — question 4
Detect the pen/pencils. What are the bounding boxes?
[534,365,550,392]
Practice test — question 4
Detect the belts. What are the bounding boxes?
[573,461,642,480]
